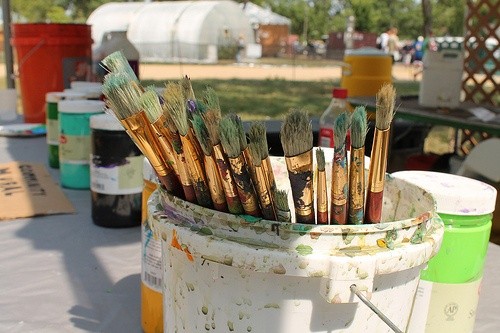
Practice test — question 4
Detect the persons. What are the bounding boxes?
[375,28,439,80]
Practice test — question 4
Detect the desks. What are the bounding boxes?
[0,88,500,333]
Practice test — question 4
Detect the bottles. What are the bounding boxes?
[93,31,139,84]
[318,88,354,152]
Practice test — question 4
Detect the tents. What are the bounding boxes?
[237,1,292,42]
[85,0,255,63]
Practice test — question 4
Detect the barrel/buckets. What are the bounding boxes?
[10,23,95,124]
[141,155,445,333]
[419,49,465,109]
[339,49,392,120]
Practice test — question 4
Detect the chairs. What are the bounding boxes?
[455,138,500,246]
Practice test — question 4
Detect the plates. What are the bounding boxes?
[0,123,47,137]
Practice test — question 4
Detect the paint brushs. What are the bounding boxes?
[100,48,291,223]
[280,111,328,224]
[331,86,396,225]
